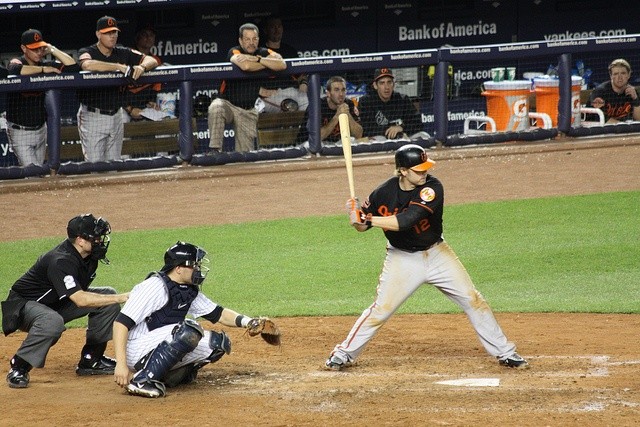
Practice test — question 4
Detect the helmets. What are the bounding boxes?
[167,241,211,286]
[67,214,113,266]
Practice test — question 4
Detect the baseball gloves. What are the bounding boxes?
[248,316,282,347]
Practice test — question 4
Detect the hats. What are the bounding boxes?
[373,69,396,82]
[22,29,49,49]
[395,144,438,173]
[97,16,121,34]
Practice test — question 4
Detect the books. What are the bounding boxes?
[137,107,177,120]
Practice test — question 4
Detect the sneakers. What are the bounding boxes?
[7,368,31,389]
[75,355,118,376]
[126,378,161,400]
[496,352,530,371]
[325,351,354,373]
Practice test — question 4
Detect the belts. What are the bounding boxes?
[10,124,45,131]
[86,106,120,116]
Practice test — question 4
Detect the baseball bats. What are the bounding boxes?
[338,113,355,201]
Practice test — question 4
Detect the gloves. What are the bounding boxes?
[345,197,363,210]
[349,210,373,228]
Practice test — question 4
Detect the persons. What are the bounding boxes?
[79,16,160,163]
[293,75,363,144]
[355,67,423,136]
[324,143,531,370]
[253,18,311,113]
[113,242,260,398]
[4,213,132,389]
[117,26,161,120]
[206,23,287,153]
[5,27,80,167]
[586,59,640,122]
